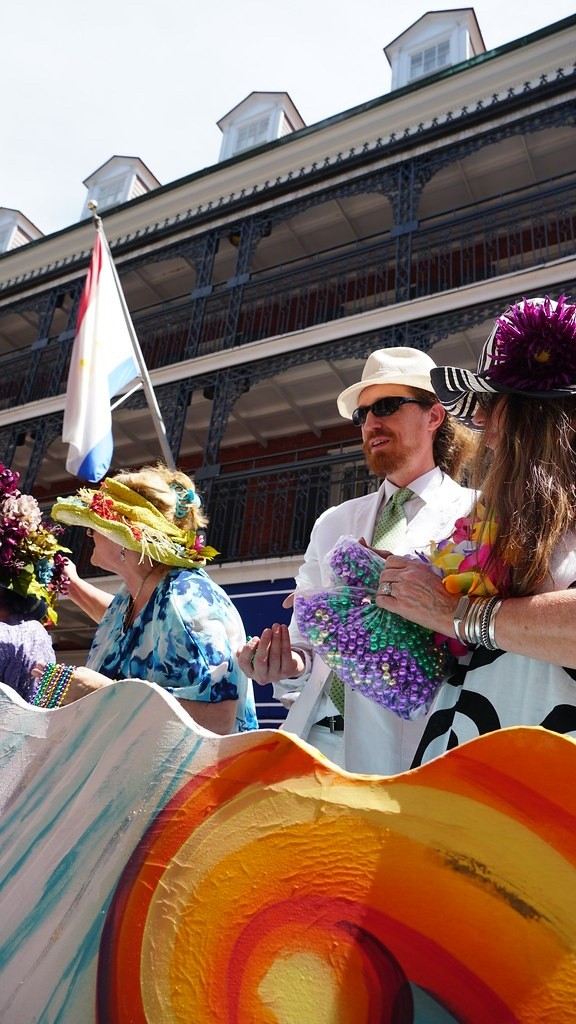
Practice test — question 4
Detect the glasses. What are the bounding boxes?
[474,392,498,409]
[90,528,95,536]
[352,396,430,427]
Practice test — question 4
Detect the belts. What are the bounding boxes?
[315,715,344,733]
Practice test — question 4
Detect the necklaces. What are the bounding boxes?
[32,663,77,709]
[132,563,158,601]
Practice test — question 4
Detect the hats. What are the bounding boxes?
[0,463,73,629]
[337,347,455,421]
[430,297,576,433]
[51,477,221,568]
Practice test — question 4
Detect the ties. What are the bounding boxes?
[328,488,415,721]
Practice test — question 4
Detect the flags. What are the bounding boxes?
[63,230,141,484]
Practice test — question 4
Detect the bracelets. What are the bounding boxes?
[453,595,503,651]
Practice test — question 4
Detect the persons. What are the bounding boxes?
[0,583,56,702]
[374,293,576,771]
[32,466,259,735]
[236,347,482,777]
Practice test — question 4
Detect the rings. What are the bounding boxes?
[382,582,392,596]
